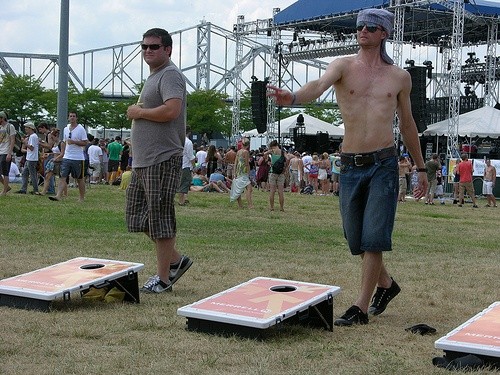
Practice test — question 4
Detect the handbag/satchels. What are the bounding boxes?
[9,162,20,177]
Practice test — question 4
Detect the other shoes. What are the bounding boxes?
[39,192,44,195]
[14,190,26,194]
[458,204,462,207]
[473,205,478,208]
[90,181,93,184]
[93,181,98,183]
[442,201,445,205]
[49,196,60,200]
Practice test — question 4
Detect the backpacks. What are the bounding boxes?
[270,155,287,174]
[7,124,21,153]
[301,186,313,195]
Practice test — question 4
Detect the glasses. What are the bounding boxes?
[356,21,382,33]
[69,131,71,139]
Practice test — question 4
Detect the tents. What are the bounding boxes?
[243,111,349,142]
[425,105,500,158]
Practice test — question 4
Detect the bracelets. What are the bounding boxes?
[415,167,427,173]
[291,93,297,108]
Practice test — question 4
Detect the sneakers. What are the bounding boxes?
[169,255,193,285]
[138,275,172,294]
[368,277,401,316]
[334,305,369,326]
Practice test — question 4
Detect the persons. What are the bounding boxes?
[122,27,195,293]
[263,9,426,325]
[0,109,498,213]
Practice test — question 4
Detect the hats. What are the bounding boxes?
[0,111,7,119]
[24,122,37,130]
